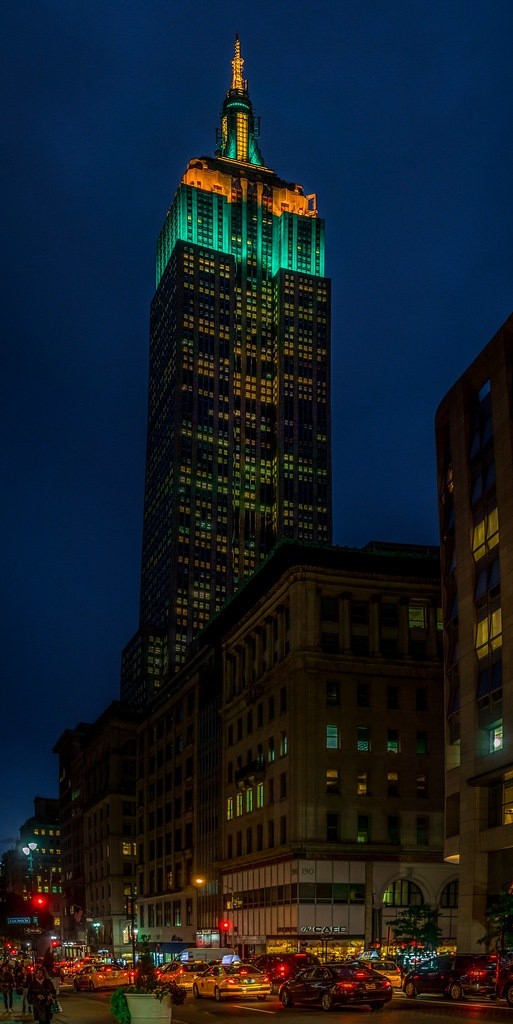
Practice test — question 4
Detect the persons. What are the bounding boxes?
[0,959,57,1024]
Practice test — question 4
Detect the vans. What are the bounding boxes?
[173,947,235,962]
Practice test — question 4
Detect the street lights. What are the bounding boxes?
[21,843,37,965]
[196,878,234,948]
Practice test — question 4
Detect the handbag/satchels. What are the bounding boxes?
[49,994,63,1013]
[16,986,23,995]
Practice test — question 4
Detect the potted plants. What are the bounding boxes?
[110,934,186,1024]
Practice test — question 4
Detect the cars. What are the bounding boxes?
[361,960,403,992]
[73,962,130,993]
[193,954,271,1002]
[401,954,500,1001]
[155,954,211,993]
[495,952,513,1007]
[278,962,393,1012]
[24,941,176,983]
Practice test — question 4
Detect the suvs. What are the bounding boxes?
[249,952,321,991]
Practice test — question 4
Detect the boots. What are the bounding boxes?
[28,1006,32,1013]
[23,1005,26,1012]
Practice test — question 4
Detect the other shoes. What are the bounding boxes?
[8,1009,13,1012]
[3,1009,8,1013]
